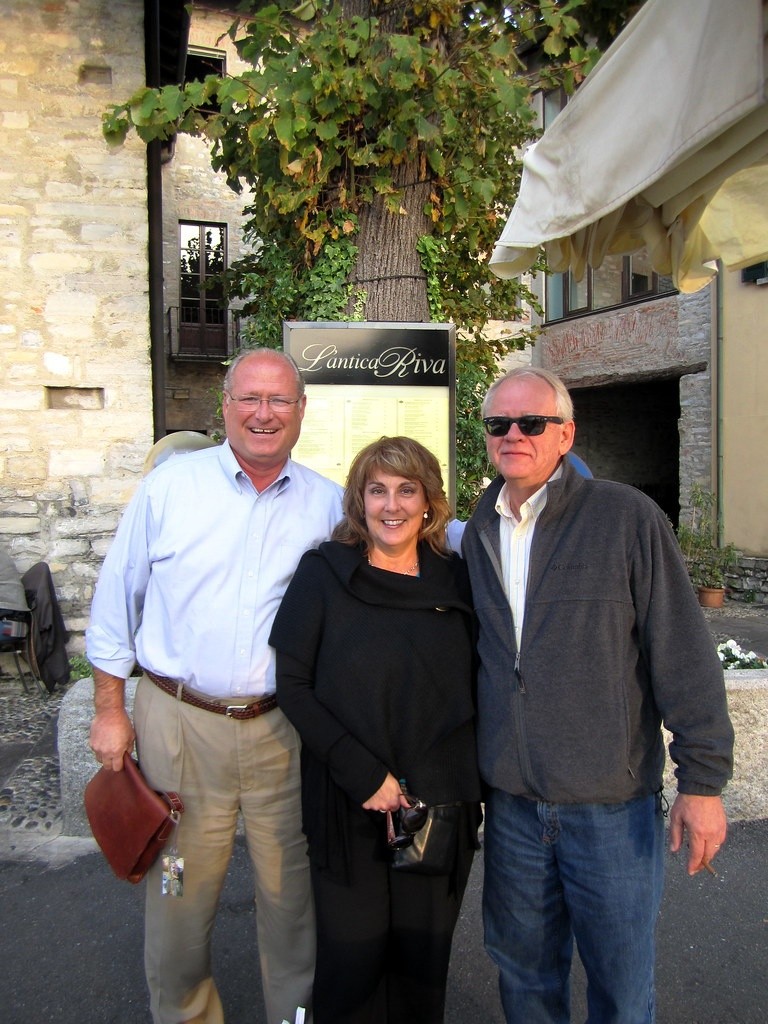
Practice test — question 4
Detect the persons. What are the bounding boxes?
[455,368,736,1024]
[0,543,31,652]
[273,436,482,1023]
[86,348,470,1024]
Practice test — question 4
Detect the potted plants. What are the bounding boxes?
[682,490,739,607]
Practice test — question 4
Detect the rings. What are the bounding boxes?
[380,809,386,812]
[715,844,721,848]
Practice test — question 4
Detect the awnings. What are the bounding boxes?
[488,0,768,293]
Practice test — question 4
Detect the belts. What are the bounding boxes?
[143,668,278,720]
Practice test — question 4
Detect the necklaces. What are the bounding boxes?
[367,551,420,575]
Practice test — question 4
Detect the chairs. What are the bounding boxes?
[0,561,71,701]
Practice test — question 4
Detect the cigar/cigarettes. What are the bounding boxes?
[687,842,718,878]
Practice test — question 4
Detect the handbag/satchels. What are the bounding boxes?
[85,751,184,884]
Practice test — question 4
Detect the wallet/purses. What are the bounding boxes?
[391,802,461,875]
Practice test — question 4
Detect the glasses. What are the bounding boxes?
[387,794,429,850]
[482,414,564,436]
[226,389,304,412]
